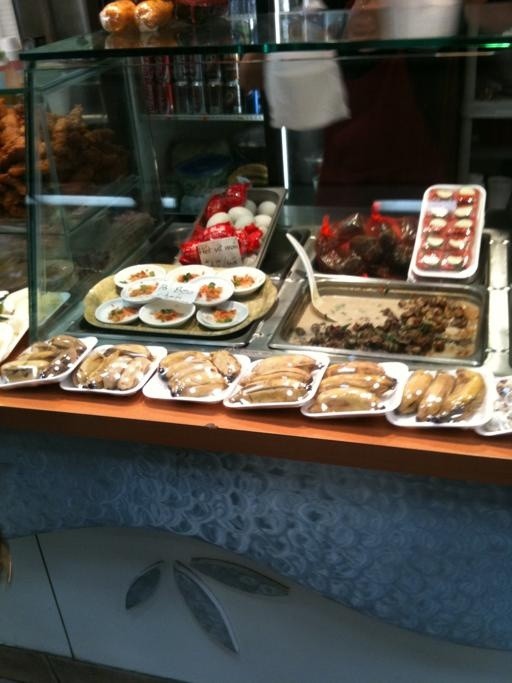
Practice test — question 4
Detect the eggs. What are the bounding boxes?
[206,197,277,265]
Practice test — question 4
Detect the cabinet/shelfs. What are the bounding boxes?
[457,47,509,215]
[0,12,511,486]
[124,0,291,208]
[0,61,142,240]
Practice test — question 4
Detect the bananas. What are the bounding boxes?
[15,335,86,379]
[232,355,321,409]
[160,347,239,396]
[310,362,397,413]
[75,343,152,388]
[401,368,485,422]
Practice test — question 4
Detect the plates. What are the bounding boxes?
[222,267,265,297]
[385,367,493,429]
[113,264,167,288]
[95,297,141,324]
[0,291,71,327]
[59,345,168,396]
[0,325,30,362]
[165,264,213,286]
[472,375,512,437]
[300,361,408,421]
[410,183,486,280]
[188,276,234,307]
[222,352,331,410]
[142,352,251,404]
[196,300,249,329]
[139,299,196,328]
[0,336,98,390]
[120,277,164,304]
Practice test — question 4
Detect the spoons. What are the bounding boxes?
[286,230,340,322]
